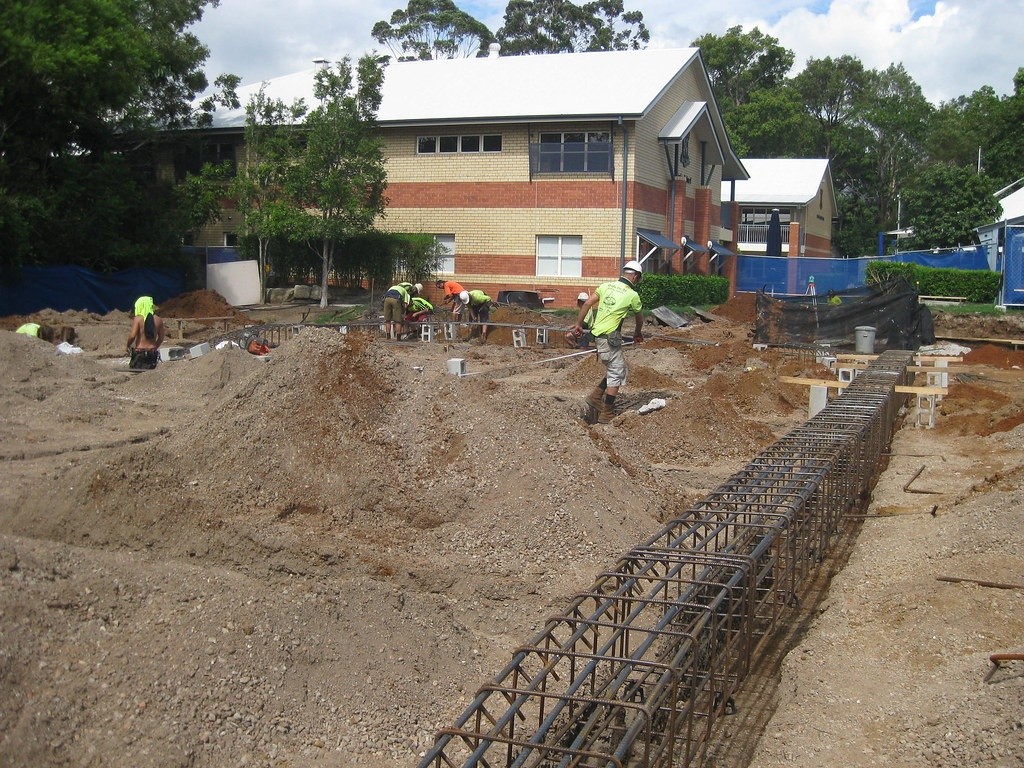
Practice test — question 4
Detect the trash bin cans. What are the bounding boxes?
[855,326,878,355]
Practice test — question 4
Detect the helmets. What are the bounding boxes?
[623,261,644,282]
[577,293,588,301]
[415,283,423,294]
[459,291,470,305]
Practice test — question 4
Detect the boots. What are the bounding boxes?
[597,404,616,424]
[586,386,604,413]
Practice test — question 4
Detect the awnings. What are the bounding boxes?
[638,231,682,269]
[709,242,736,270]
[684,239,709,271]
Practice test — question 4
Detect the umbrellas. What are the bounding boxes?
[767,209,782,257]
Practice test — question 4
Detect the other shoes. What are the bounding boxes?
[481,336,486,344]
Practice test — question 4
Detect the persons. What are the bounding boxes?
[436,280,464,312]
[827,289,842,306]
[384,286,410,341]
[460,289,492,342]
[398,282,424,296]
[127,296,164,372]
[565,292,593,349]
[575,261,643,424]
[16,323,53,340]
[405,297,433,320]
